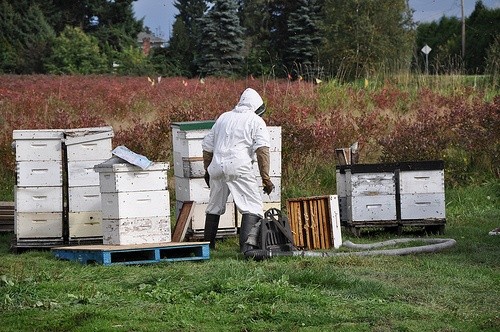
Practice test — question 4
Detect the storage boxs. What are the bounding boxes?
[12,120,282,246]
[286,160,446,248]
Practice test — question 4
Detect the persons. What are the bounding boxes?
[202,87,275,255]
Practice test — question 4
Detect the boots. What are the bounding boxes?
[204,213,220,250]
[240,214,260,253]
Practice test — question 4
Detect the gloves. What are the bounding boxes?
[255,146,275,195]
[202,150,214,188]
